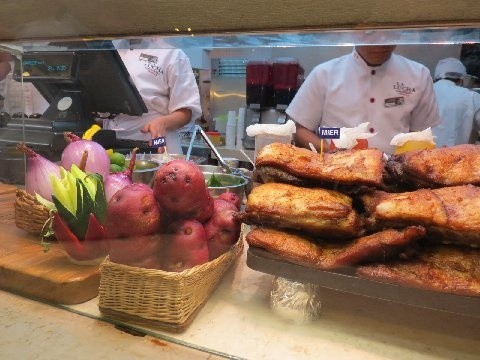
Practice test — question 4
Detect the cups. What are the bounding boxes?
[224,110,236,150]
[236,107,247,147]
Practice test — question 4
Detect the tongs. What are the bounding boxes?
[185,125,232,174]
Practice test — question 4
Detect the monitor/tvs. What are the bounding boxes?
[0,39,148,132]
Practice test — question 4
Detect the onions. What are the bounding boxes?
[105,145,136,201]
[18,144,58,201]
[62,132,110,178]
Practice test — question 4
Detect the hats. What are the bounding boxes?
[433,57,467,81]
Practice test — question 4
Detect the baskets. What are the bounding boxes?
[96,223,246,334]
[14,189,55,238]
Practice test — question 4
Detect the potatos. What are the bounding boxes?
[207,198,240,258]
[109,182,159,267]
[164,220,208,270]
[152,156,205,214]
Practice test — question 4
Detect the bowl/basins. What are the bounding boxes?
[199,164,244,175]
[123,158,162,188]
[128,153,206,165]
[204,171,248,197]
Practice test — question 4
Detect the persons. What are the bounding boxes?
[429,56,480,149]
[0,45,52,118]
[93,37,202,155]
[283,29,441,155]
